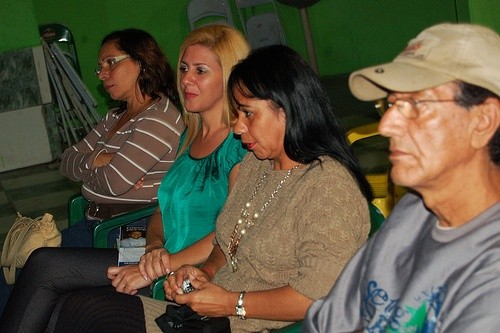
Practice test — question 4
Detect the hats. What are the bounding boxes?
[348,22,500,100]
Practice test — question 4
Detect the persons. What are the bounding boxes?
[0,29,187,317]
[0,24,252,333]
[304,23,500,333]
[53,43,377,333]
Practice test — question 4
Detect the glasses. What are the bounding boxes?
[95,54,130,74]
[374,98,460,119]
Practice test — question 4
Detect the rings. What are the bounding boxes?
[167,271,174,278]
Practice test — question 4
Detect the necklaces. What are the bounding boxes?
[227,159,301,274]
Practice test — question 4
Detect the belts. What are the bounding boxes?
[85,202,148,220]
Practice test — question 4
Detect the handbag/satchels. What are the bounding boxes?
[0,211,62,283]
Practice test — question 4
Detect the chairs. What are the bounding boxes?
[67,193,385,333]
[236,0,288,51]
[188,0,233,40]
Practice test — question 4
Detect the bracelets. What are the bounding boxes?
[235,290,247,320]
